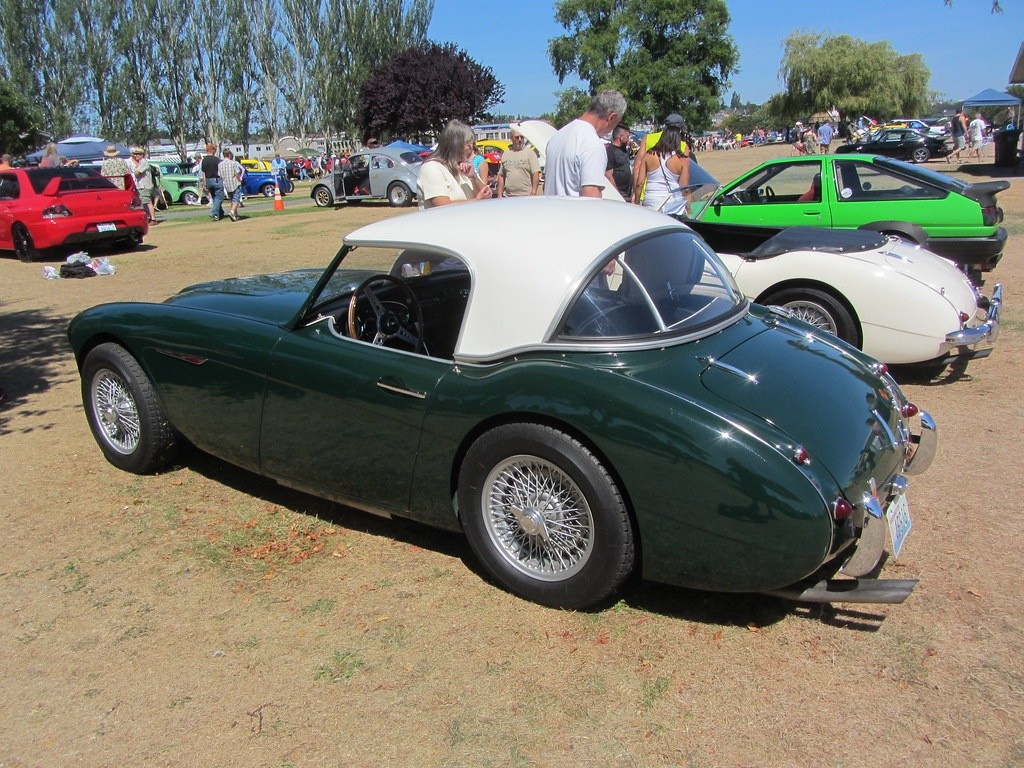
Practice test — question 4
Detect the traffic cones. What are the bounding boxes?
[272,181,285,211]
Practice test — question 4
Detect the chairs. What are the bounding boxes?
[796,173,822,202]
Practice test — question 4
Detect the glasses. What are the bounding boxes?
[513,136,523,140]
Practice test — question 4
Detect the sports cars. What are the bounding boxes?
[609,181,1004,371]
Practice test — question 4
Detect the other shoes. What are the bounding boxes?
[219,211,224,218]
[209,214,218,220]
[206,202,213,206]
[154,206,160,212]
[229,212,237,221]
[194,202,201,205]
[149,219,158,225]
[946,155,951,164]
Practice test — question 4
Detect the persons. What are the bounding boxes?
[605,125,632,202]
[416,120,493,208]
[633,115,690,219]
[690,125,771,153]
[201,143,225,221]
[498,129,541,198]
[470,143,488,184]
[254,157,265,169]
[127,148,159,225]
[340,153,347,163]
[360,139,375,167]
[786,118,863,153]
[544,90,627,290]
[100,145,130,190]
[946,109,987,164]
[310,153,327,180]
[272,153,286,170]
[39,142,79,167]
[0,154,11,169]
[218,148,245,221]
[298,157,311,182]
[192,153,213,207]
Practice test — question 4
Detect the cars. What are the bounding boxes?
[0,161,151,263]
[833,115,955,164]
[685,151,1011,276]
[416,138,546,183]
[64,193,938,613]
[146,152,349,210]
[309,147,425,209]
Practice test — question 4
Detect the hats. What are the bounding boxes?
[665,113,688,131]
[133,148,145,154]
[104,146,119,156]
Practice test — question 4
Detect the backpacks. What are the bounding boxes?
[147,164,159,187]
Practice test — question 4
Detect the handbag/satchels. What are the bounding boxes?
[44,252,116,278]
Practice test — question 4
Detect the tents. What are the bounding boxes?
[386,140,428,153]
[26,132,131,167]
[961,88,1021,129]
